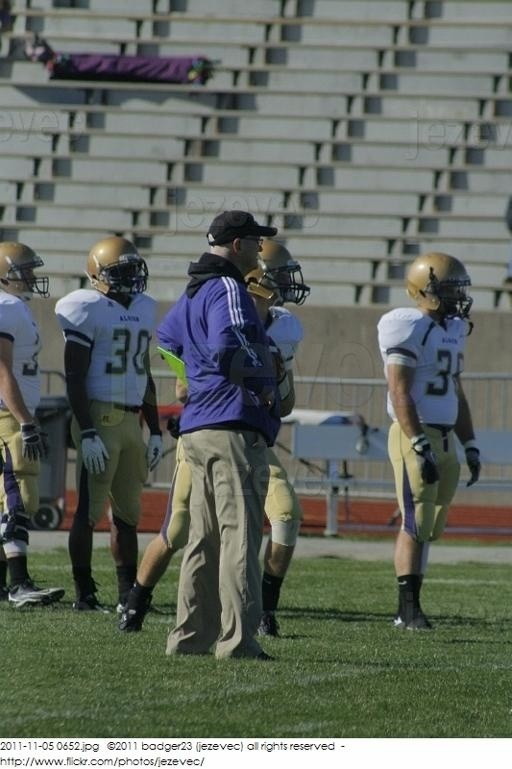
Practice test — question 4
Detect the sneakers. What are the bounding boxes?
[8,578,280,662]
[394,612,433,632]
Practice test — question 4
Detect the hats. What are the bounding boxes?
[205,210,279,246]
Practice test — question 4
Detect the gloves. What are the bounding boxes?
[464,441,482,487]
[20,421,48,462]
[146,433,161,471]
[411,435,440,484]
[81,427,110,475]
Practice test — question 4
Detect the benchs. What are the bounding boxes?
[267,421,511,538]
[2,1,512,315]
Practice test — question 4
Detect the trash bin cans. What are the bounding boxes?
[24,395,73,530]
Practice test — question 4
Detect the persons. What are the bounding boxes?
[375,251,483,633]
[116,239,311,640]
[57,235,165,615]
[0,238,68,609]
[155,209,286,662]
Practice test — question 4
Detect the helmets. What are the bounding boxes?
[0,243,50,300]
[85,238,148,300]
[245,240,310,306]
[406,254,474,320]
